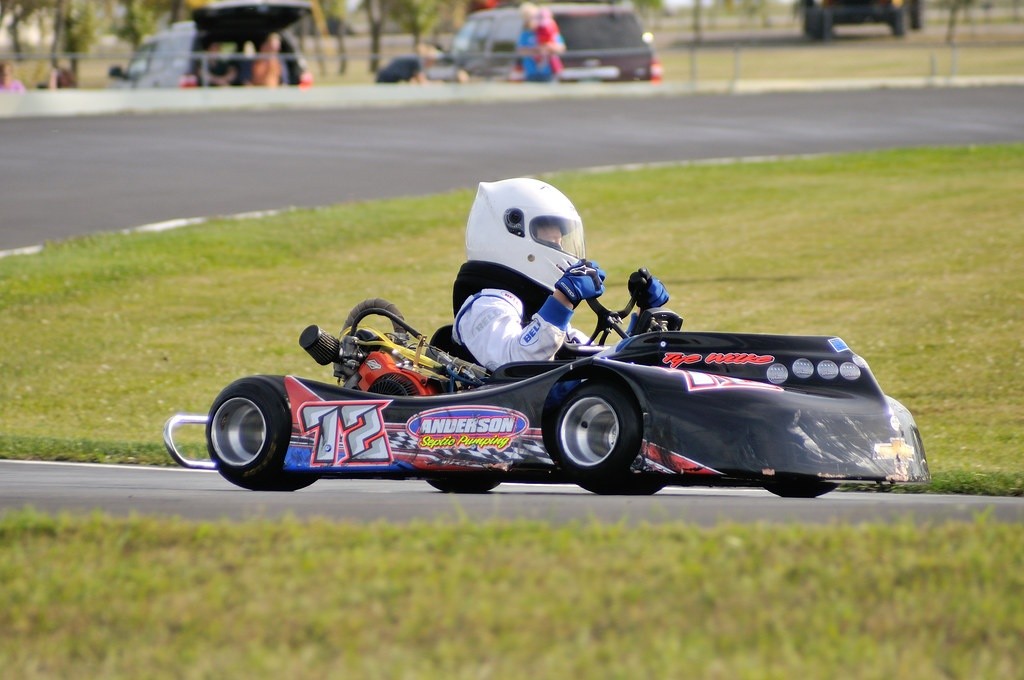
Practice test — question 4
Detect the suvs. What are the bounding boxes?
[430,5,660,85]
[107,1,317,88]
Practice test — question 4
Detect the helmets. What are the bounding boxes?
[465,177,586,293]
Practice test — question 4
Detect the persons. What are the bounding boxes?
[451,178,670,376]
[240,33,290,86]
[199,44,238,87]
[517,2,566,81]
[376,44,439,84]
[0,61,24,92]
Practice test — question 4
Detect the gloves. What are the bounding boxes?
[554,258,606,310]
[636,267,670,311]
